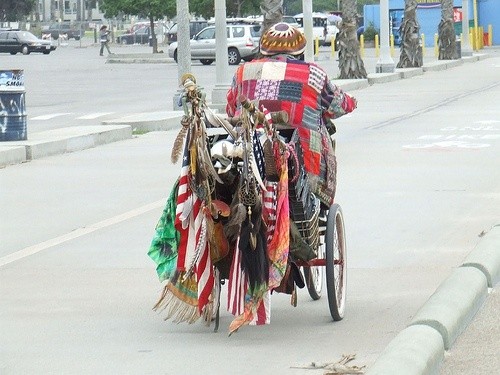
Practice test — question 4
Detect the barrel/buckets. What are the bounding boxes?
[0,69,28,141]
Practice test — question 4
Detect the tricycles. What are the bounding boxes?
[181,73,347,334]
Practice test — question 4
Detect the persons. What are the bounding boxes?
[98,25,111,56]
[227,21,358,216]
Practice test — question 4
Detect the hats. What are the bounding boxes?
[259,23,307,56]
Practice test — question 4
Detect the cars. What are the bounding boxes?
[0,31,57,55]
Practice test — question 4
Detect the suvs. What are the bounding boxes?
[41,23,86,40]
[117,11,343,61]
[167,24,264,65]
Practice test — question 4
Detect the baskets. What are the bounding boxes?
[288,136,319,251]
